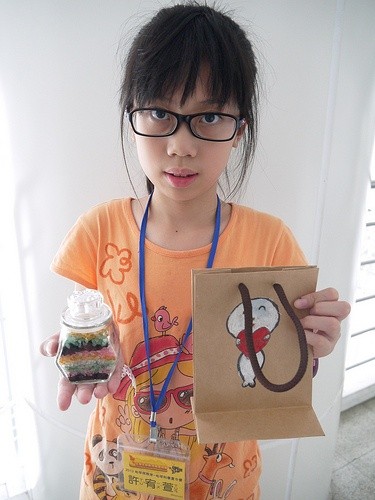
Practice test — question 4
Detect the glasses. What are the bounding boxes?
[124,98,247,144]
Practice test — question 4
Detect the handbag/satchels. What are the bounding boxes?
[189,265,329,445]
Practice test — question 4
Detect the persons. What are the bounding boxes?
[39,0,349,500]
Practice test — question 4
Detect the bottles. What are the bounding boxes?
[56,289,121,385]
